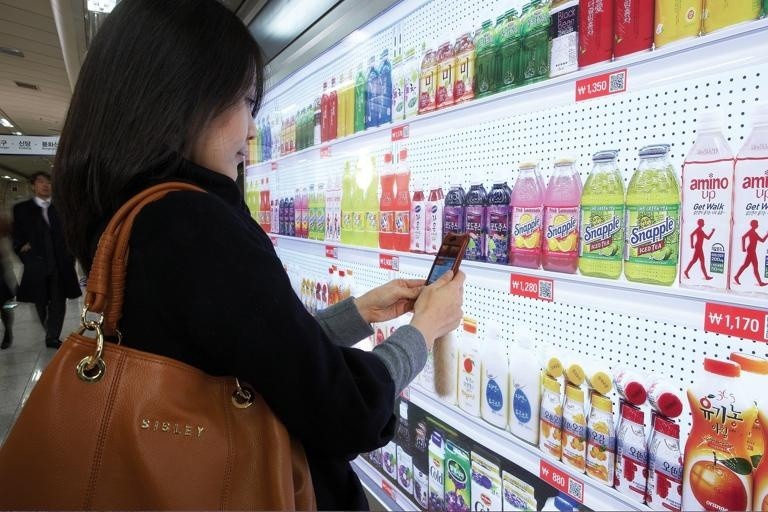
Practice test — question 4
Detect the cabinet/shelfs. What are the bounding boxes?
[245,17,768,512]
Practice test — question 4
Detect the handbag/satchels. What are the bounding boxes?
[0,324,322,512]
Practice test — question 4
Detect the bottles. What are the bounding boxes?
[243,1,767,317]
[381,316,767,512]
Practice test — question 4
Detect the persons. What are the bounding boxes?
[1,213,19,352]
[11,171,84,350]
[53,2,468,512]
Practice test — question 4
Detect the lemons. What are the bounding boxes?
[515,213,577,252]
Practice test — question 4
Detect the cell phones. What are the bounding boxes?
[424,232,470,286]
